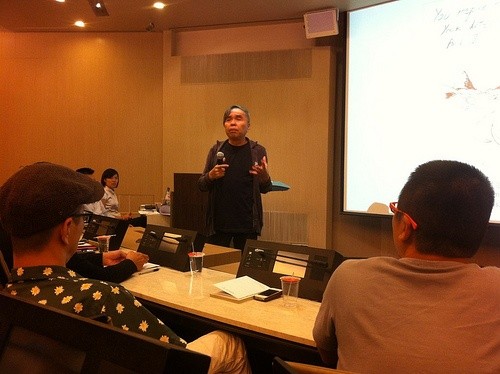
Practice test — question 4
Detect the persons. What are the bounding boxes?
[64,168,147,283]
[312,159,500,374]
[0,163,253,374]
[198,105,273,252]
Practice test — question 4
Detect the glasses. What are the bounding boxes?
[62,210,93,223]
[389,202,417,230]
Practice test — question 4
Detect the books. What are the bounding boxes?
[210,276,270,304]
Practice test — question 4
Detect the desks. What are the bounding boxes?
[97,244,321,352]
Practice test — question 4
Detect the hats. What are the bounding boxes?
[0,161,104,229]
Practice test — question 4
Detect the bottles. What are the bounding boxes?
[165,187,171,206]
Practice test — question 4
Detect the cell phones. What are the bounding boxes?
[254,287,282,301]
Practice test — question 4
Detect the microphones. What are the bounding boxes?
[216,150,224,165]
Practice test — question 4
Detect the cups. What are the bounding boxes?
[97,235,111,252]
[188,251,205,276]
[280,275,302,307]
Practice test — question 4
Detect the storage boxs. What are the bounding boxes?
[159,205,170,213]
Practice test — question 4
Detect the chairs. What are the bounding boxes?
[0,291,211,374]
